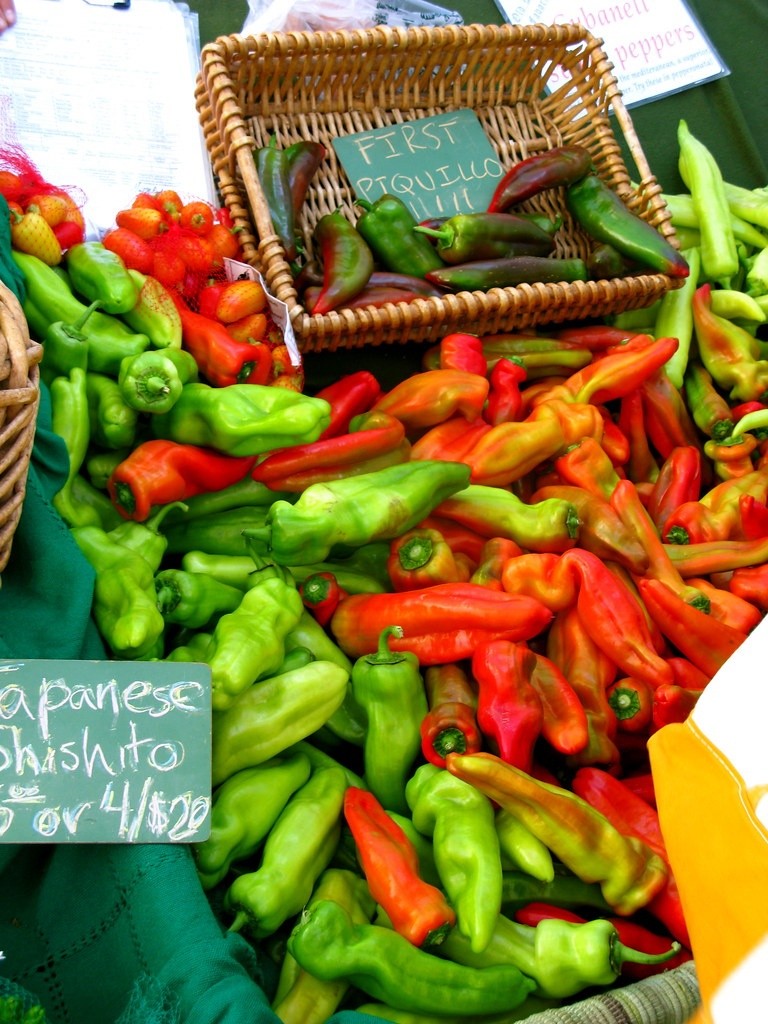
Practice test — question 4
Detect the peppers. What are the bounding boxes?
[0,118,768,1024]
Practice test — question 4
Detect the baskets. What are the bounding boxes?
[194,21,686,355]
[0,280,44,572]
